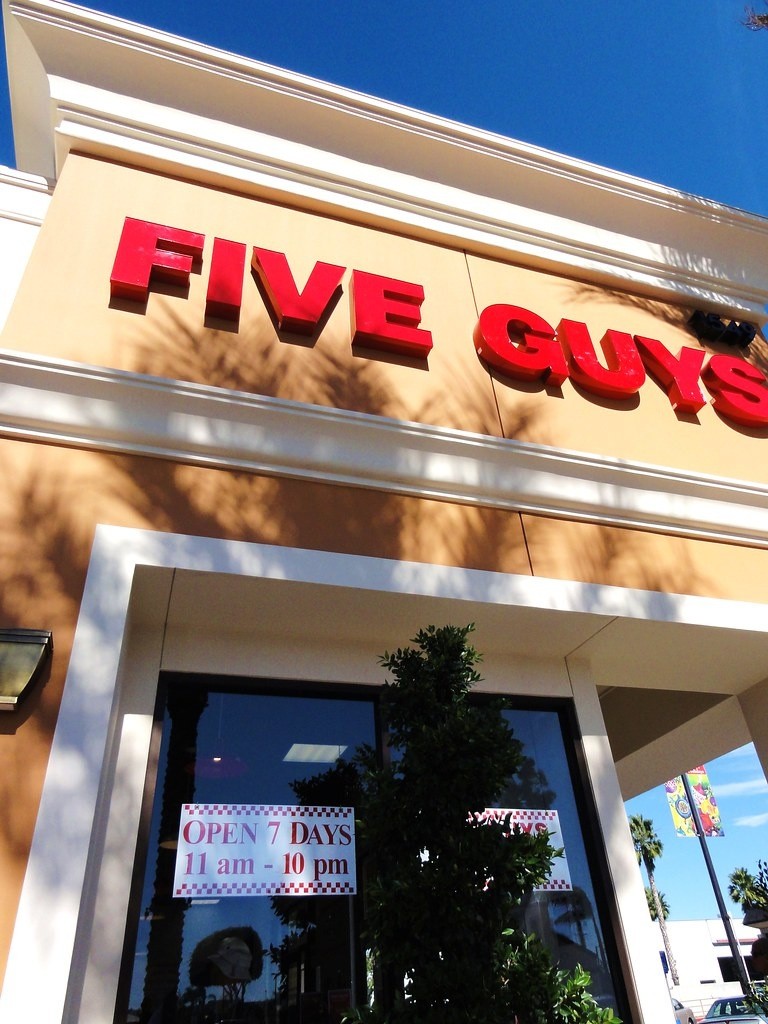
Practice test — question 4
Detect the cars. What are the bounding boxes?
[670,998,697,1024]
[695,996,768,1024]
